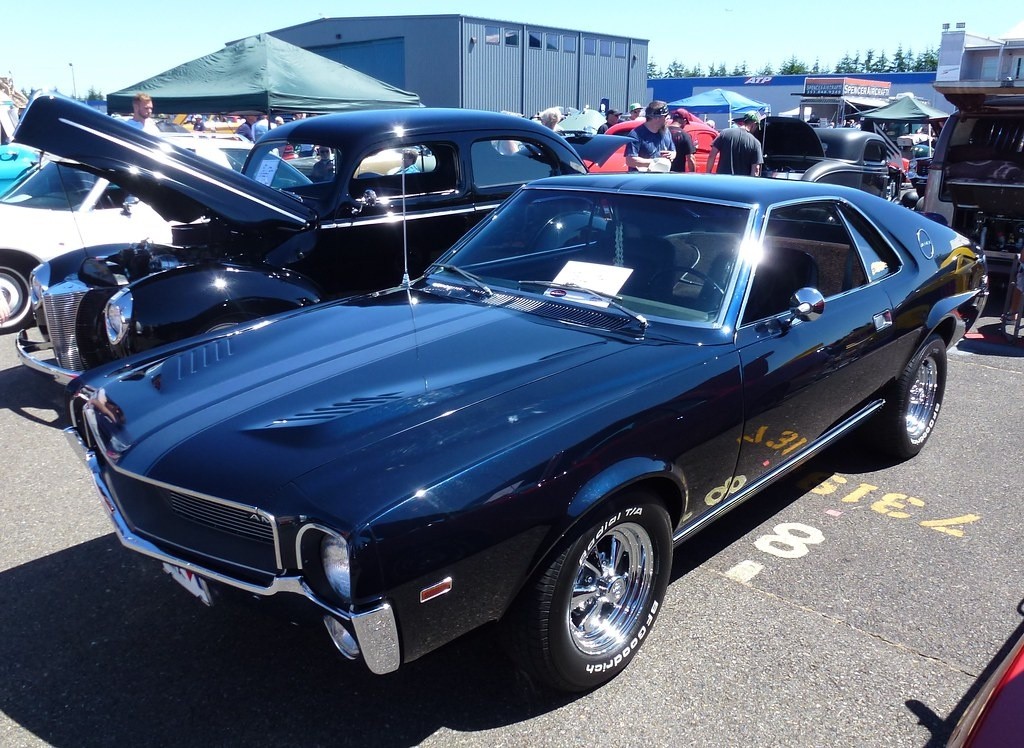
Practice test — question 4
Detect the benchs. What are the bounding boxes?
[668,231,867,303]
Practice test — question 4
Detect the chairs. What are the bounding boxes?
[589,235,677,301]
[697,247,820,324]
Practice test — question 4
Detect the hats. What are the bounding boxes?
[744,110,761,130]
[605,109,623,116]
[629,103,643,112]
[674,108,690,125]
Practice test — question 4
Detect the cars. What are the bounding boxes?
[0,92,639,394]
[579,91,1024,275]
[65,172,989,697]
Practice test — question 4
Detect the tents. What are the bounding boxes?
[778,105,813,123]
[857,94,951,164]
[666,88,771,130]
[106,32,423,134]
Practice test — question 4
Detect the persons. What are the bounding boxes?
[596,109,623,135]
[624,100,677,173]
[506,107,562,157]
[395,148,421,177]
[123,92,161,133]
[665,108,696,173]
[625,103,644,121]
[310,147,336,184]
[188,111,320,157]
[705,109,764,177]
